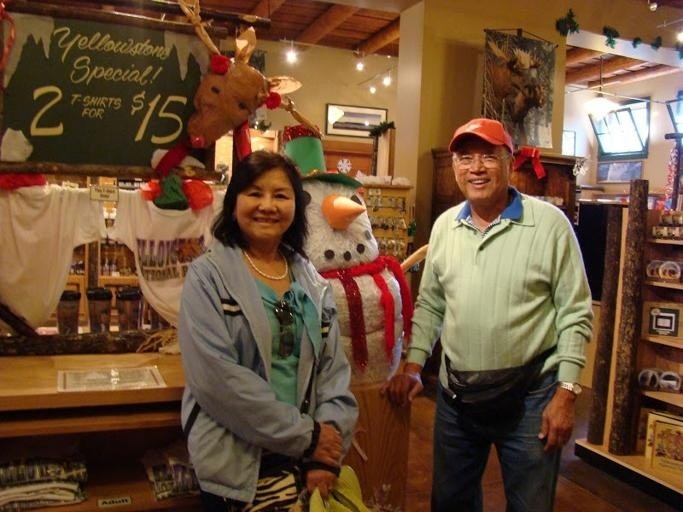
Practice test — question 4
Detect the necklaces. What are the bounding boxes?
[243,249,289,280]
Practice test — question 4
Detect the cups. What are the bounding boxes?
[284,137,327,173]
[646,195,656,210]
[651,211,682,237]
[355,169,411,187]
[56,285,143,335]
[532,196,563,206]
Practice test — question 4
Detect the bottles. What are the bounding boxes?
[68,243,134,277]
[675,191,683,210]
[363,187,419,273]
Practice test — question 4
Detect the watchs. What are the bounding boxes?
[560,382,583,396]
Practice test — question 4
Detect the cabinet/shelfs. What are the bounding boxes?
[628,234,683,459]
[428,145,581,236]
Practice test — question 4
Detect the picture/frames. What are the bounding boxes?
[325,102,388,138]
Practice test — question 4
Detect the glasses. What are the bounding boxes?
[451,154,504,169]
[274,300,294,356]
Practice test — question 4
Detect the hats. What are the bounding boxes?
[310,465,370,512]
[449,118,513,154]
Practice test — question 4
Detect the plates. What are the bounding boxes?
[576,198,617,204]
[647,259,683,280]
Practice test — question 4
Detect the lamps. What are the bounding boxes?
[587,55,612,119]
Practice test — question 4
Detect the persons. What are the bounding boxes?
[379,118,594,512]
[178,149,361,512]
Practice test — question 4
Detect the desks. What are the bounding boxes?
[0,351,211,511]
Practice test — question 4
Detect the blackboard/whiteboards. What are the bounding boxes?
[0,3,227,180]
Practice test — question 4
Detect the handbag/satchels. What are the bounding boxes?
[441,365,540,418]
